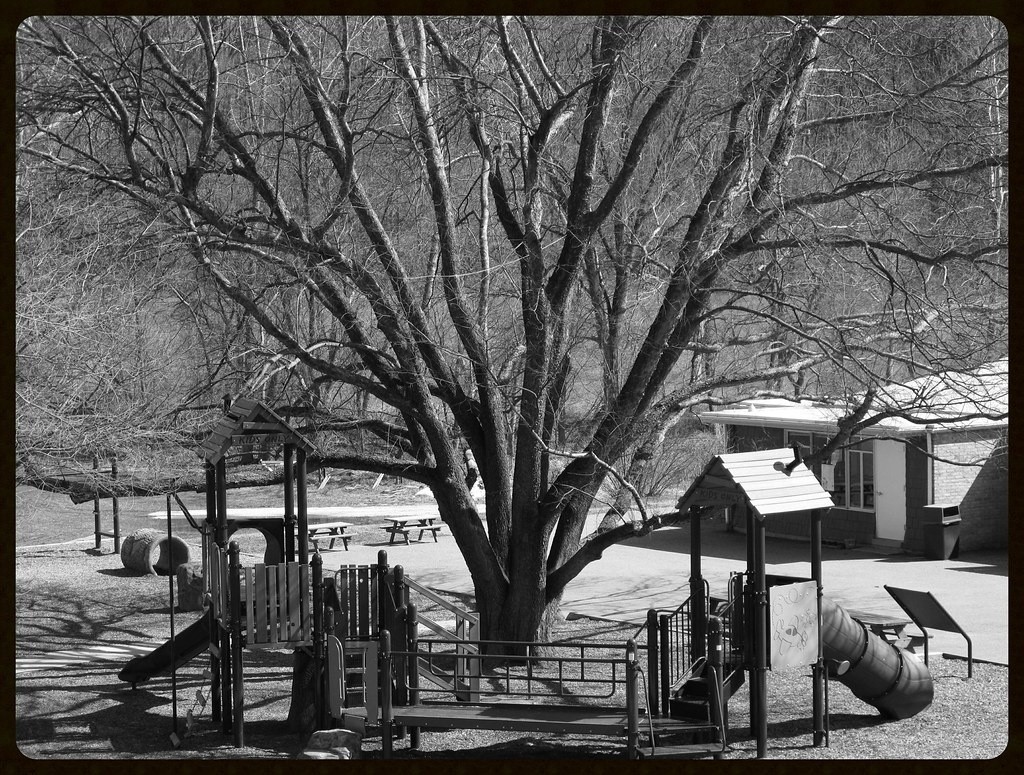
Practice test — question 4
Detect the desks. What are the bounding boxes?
[383,517,436,545]
[845,608,916,655]
[294,521,354,549]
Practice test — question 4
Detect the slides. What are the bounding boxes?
[818,592,935,716]
[118,610,224,686]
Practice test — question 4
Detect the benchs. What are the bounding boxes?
[379,523,428,544]
[868,627,934,654]
[399,525,441,545]
[307,534,352,556]
[294,532,332,554]
